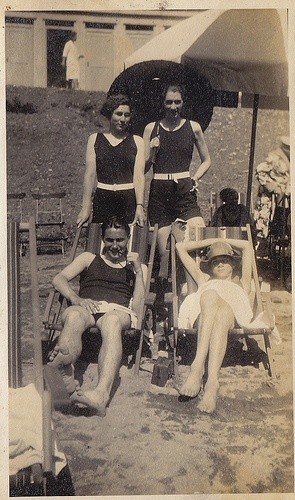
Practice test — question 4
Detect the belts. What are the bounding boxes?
[96,181,135,192]
[153,170,190,181]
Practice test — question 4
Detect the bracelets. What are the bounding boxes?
[136,204,145,208]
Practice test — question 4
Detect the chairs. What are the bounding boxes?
[167,223,274,376]
[44,227,159,376]
[7,192,29,256]
[32,193,66,254]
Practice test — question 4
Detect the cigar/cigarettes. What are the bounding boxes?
[193,185,199,192]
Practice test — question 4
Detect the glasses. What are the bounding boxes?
[210,258,232,264]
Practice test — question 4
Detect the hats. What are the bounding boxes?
[199,241,242,274]
[279,133,291,148]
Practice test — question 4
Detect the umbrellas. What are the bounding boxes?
[121,8,289,211]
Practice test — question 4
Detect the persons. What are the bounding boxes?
[77,95,148,228]
[256,135,290,259]
[175,237,254,412]
[47,218,145,415]
[210,188,256,239]
[144,80,210,257]
[61,30,81,90]
